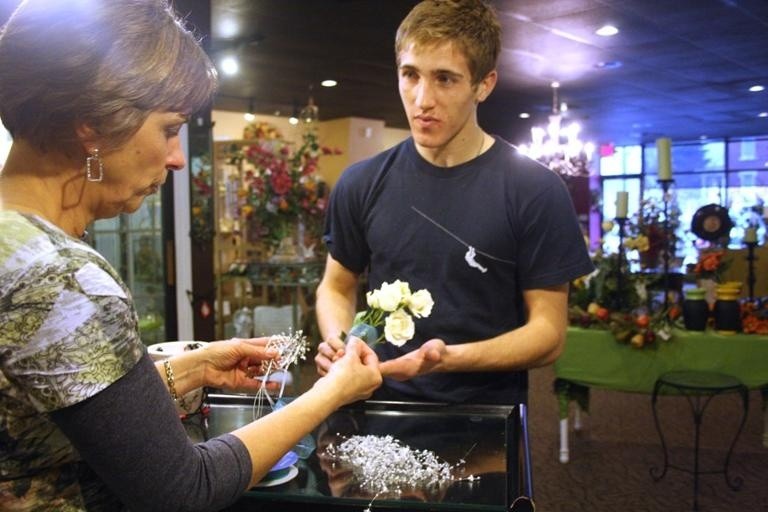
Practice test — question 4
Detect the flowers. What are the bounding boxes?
[216,119,344,261]
[352,277,434,349]
[567,199,767,347]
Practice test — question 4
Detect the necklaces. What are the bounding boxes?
[477,127,486,158]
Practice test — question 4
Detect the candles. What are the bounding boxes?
[656,137,671,179]
[615,191,628,218]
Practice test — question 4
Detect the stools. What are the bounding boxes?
[650,368,749,510]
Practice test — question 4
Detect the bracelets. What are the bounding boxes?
[163,359,183,400]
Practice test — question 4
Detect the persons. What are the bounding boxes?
[0,1,385,512]
[311,1,597,406]
[310,407,507,504]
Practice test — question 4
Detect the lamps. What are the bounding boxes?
[515,79,594,176]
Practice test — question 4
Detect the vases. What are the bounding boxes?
[267,235,303,272]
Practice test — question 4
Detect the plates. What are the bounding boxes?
[692,203,732,241]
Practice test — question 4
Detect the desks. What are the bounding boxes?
[551,328,768,465]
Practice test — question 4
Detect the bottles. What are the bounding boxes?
[713,281,743,335]
[683,288,709,332]
